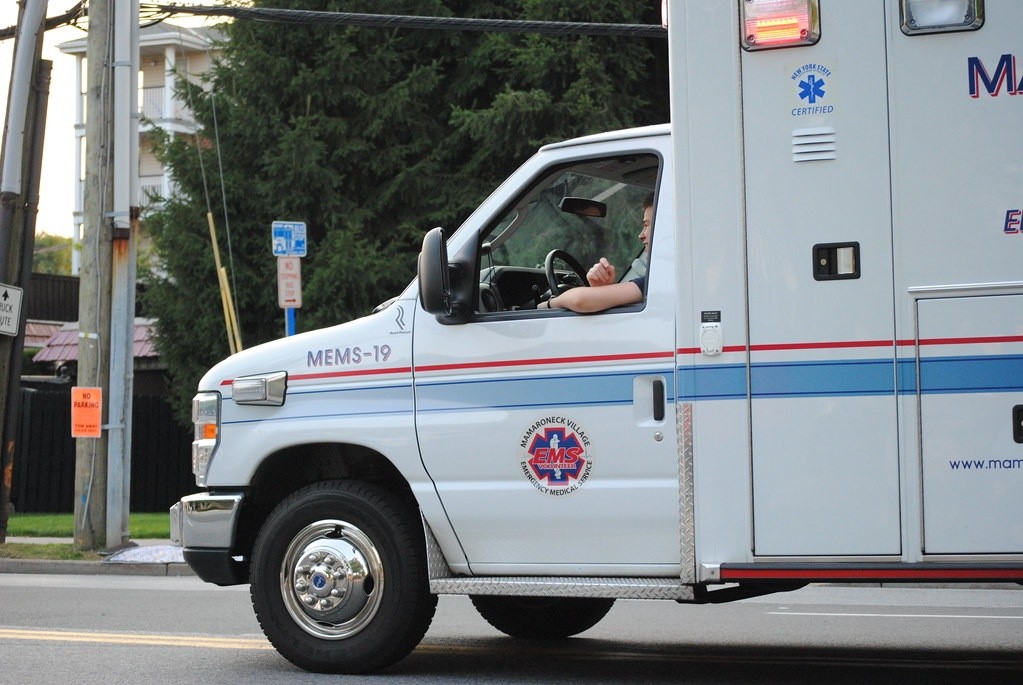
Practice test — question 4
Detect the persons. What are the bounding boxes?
[536,192,655,313]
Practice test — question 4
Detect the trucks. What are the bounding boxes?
[170,0,1023,673]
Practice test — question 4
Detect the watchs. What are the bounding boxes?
[547,295,556,308]
